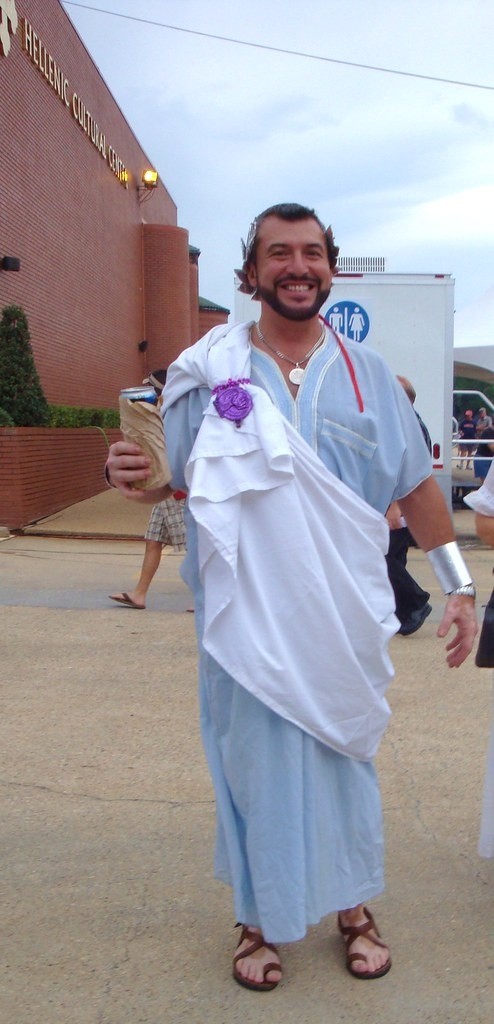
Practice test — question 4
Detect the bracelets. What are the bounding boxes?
[448,585,476,600]
[426,541,473,595]
[104,460,117,489]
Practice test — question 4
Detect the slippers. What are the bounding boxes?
[108,592,146,611]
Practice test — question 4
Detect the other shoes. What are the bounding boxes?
[466,466,473,470]
[457,465,463,470]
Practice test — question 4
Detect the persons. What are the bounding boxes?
[103,203,494,991]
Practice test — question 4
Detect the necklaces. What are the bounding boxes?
[256,320,325,385]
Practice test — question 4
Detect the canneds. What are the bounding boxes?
[118,386,173,490]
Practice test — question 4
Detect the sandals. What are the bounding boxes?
[231,918,283,992]
[337,910,392,981]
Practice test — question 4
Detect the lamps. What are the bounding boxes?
[3,257,20,272]
[139,341,148,351]
[137,167,158,203]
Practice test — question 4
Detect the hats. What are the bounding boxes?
[465,410,473,416]
[142,373,164,391]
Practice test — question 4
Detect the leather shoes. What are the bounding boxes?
[399,601,433,635]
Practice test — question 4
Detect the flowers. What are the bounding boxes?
[213,386,253,428]
